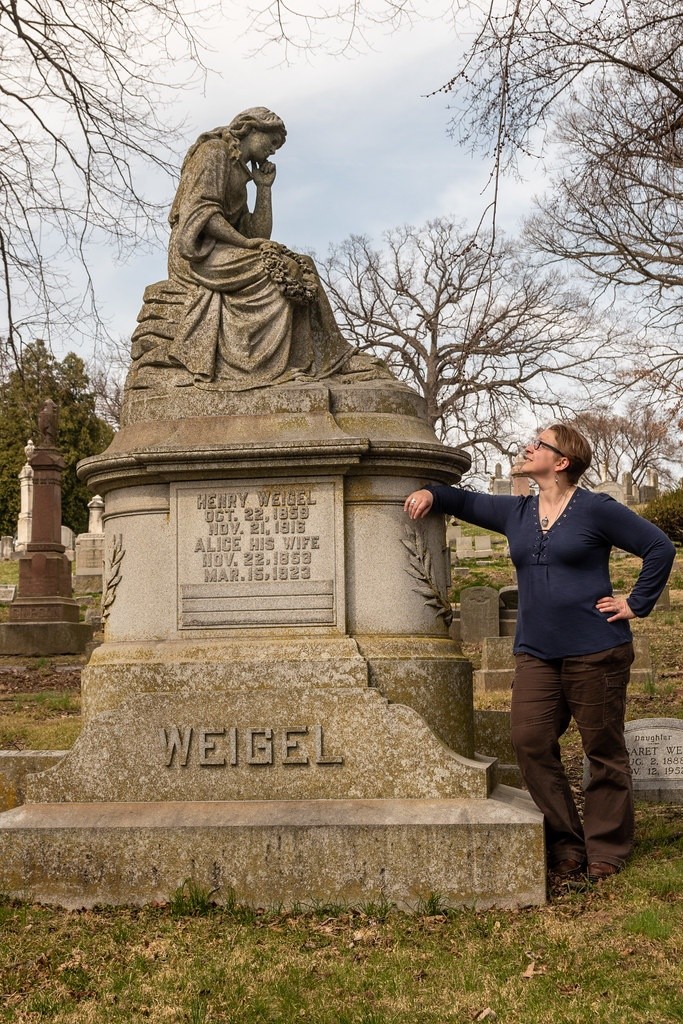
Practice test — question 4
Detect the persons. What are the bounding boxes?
[403,424,677,879]
[167,106,357,391]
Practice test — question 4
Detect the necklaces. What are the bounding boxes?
[540,490,566,526]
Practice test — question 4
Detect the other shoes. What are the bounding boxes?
[549,863,586,886]
[588,861,619,883]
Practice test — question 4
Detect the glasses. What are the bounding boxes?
[531,439,565,455]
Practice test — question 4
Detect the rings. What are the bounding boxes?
[411,498,416,504]
